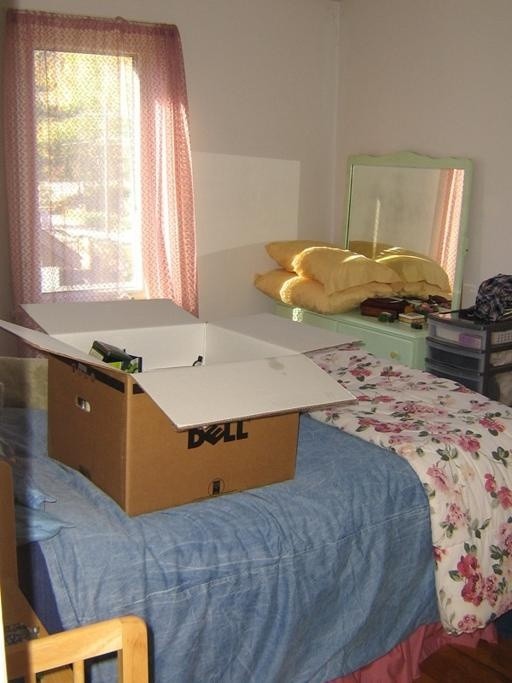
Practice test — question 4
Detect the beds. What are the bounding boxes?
[1,344,512,683]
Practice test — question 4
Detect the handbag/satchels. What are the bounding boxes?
[473,275,512,322]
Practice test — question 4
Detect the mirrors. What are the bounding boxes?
[341,154,473,318]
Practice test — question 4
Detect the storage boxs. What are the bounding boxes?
[0,298,363,517]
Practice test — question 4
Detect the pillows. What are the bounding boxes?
[391,281,446,301]
[255,268,394,315]
[348,238,451,292]
[265,239,396,291]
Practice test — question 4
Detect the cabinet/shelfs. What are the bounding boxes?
[430,320,512,403]
[272,301,427,372]
[0,458,150,683]
